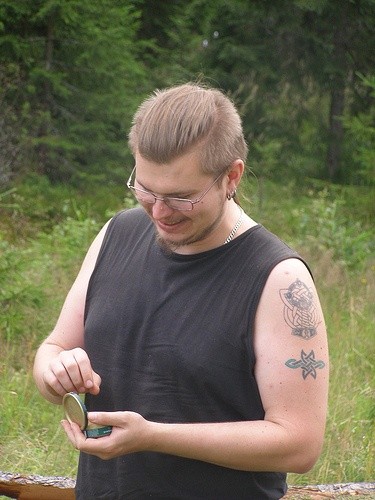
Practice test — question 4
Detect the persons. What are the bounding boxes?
[31,82,330,500]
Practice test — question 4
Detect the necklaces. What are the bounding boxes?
[225,203,244,245]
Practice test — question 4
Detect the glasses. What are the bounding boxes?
[126,164,225,212]
